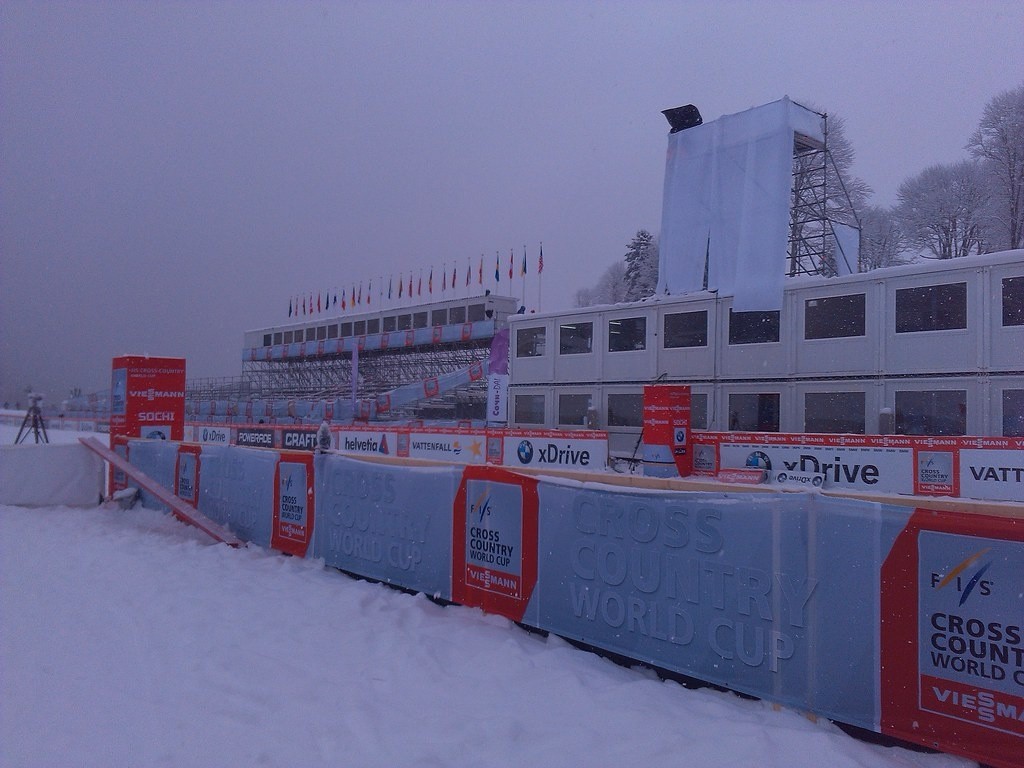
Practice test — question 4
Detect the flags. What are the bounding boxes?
[429,269,432,293]
[342,289,346,308]
[409,275,413,298]
[451,268,456,288]
[398,280,402,299]
[520,251,527,278]
[442,271,446,292]
[466,266,471,287]
[495,255,499,281]
[366,283,371,304]
[350,287,355,307]
[478,258,482,284]
[538,246,544,273]
[326,292,330,309]
[508,252,513,279]
[333,291,337,304]
[288,293,321,317]
[417,276,421,296]
[358,285,361,303]
[388,279,391,299]
